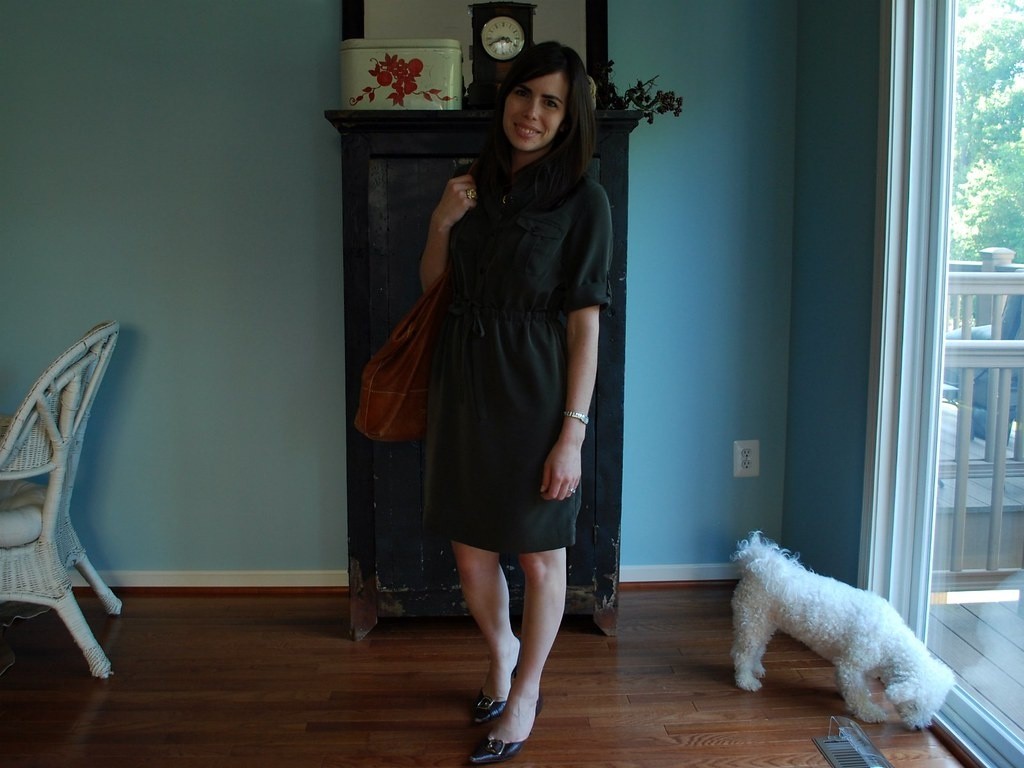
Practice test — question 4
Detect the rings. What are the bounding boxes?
[465,188,477,199]
[567,488,575,494]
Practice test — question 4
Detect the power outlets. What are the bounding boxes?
[733,439,761,478]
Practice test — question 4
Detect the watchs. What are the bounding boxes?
[562,411,589,426]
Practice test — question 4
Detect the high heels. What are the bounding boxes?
[465,687,542,766]
[471,636,522,724]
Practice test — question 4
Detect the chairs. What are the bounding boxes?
[945,269,1024,448]
[0,320,121,679]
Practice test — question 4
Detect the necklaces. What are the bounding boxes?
[503,190,513,204]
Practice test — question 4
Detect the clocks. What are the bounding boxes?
[467,0,538,108]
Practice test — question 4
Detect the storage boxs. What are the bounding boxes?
[340,38,463,111]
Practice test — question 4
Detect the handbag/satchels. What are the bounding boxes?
[355,271,448,440]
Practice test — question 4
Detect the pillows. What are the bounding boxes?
[944,324,991,384]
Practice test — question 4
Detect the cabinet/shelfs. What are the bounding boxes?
[324,108,642,641]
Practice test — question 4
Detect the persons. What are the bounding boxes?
[419,39,613,767]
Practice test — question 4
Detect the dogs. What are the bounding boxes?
[729,529,956,732]
